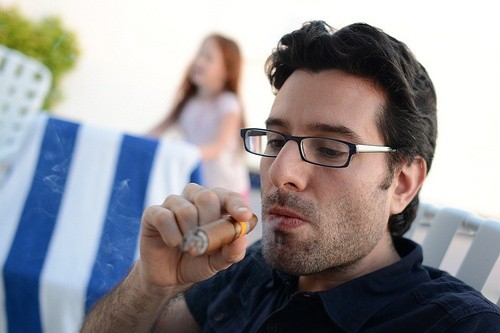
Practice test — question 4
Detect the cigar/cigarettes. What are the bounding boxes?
[176,211,259,257]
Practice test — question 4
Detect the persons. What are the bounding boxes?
[142,28,249,213]
[79,19,500,333]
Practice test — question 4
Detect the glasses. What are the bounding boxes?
[240,128,396,168]
